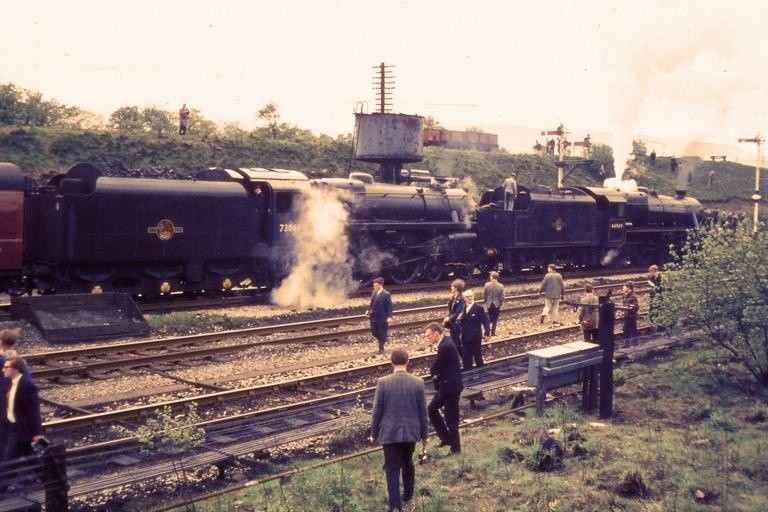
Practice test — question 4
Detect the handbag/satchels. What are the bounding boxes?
[579,316,593,328]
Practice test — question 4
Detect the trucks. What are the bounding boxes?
[422,126,499,152]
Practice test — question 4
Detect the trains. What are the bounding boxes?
[0,156,711,297]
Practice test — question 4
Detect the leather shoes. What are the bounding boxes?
[433,439,450,447]
[446,446,459,456]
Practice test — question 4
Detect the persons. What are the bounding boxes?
[622,279,639,347]
[0,327,46,496]
[533,135,570,158]
[503,173,518,211]
[538,263,564,323]
[456,290,491,371]
[444,278,465,355]
[649,149,694,184]
[367,277,393,352]
[648,264,662,321]
[700,207,744,232]
[180,104,189,134]
[483,271,505,336]
[368,350,429,511]
[707,170,715,188]
[426,321,464,456]
[578,285,599,341]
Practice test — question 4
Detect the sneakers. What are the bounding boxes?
[540,315,545,324]
